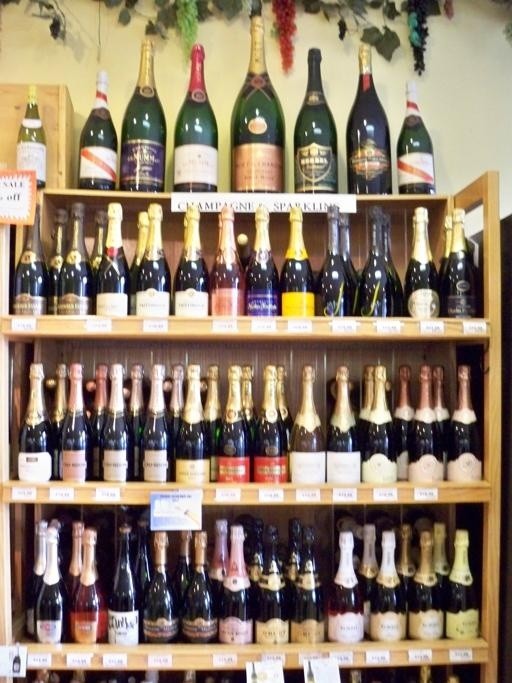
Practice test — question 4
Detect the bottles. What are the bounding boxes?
[293,47,338,192]
[78,70,118,190]
[16,361,482,484]
[16,84,48,187]
[24,511,480,641]
[120,38,166,191]
[173,43,219,191]
[396,80,435,193]
[345,43,392,194]
[10,200,484,317]
[230,15,285,191]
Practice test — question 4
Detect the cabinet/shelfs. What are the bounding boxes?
[1,170,501,683]
